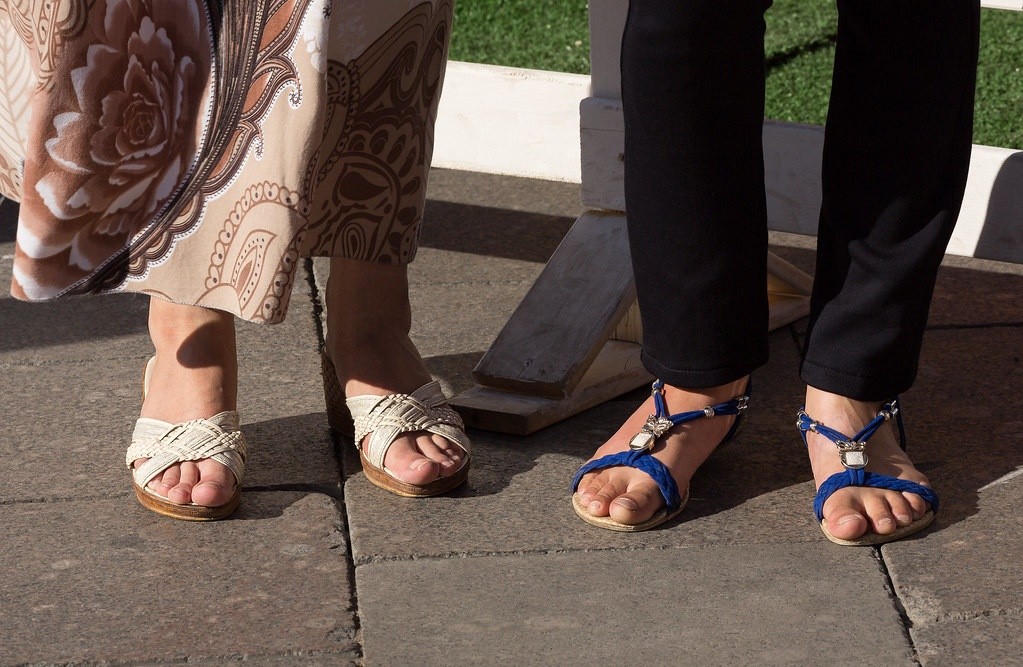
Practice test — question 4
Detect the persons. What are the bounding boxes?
[568,1,982,548]
[0,1,478,522]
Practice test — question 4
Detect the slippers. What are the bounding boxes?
[125,352,247,521]
[319,342,472,497]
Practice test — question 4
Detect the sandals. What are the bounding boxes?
[571,376,754,532]
[796,395,940,547]
